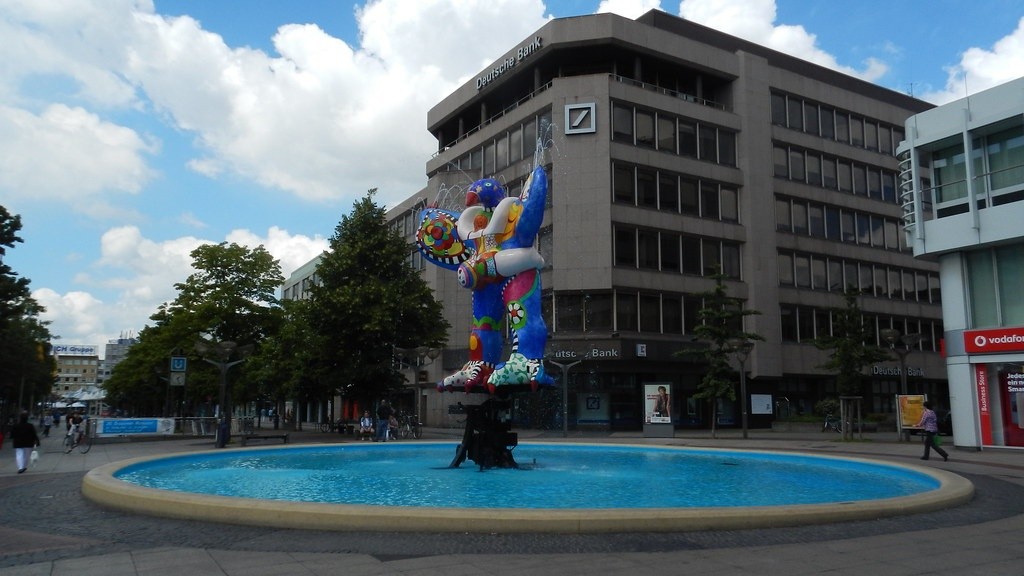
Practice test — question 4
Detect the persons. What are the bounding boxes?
[912,401,950,462]
[260,407,267,422]
[373,399,390,442]
[652,386,671,417]
[360,410,375,441]
[100,408,129,418]
[273,413,279,430]
[7,413,37,474]
[7,403,61,440]
[66,410,88,450]
[268,408,273,421]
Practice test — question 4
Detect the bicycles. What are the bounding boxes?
[320,417,348,433]
[399,410,423,439]
[821,411,849,433]
[61,424,93,454]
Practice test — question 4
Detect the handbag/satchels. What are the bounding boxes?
[933,433,942,446]
[31,444,39,467]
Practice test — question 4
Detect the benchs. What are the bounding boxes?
[355,429,398,440]
[241,432,288,446]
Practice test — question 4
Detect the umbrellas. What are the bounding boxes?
[42,401,86,409]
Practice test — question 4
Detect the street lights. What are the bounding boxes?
[195,340,256,404]
[393,345,442,438]
[881,328,923,395]
[728,335,755,439]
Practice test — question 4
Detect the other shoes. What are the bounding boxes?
[18,468,27,473]
